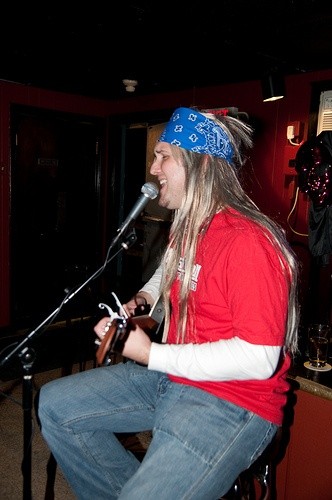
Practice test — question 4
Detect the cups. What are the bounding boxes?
[307,324,330,367]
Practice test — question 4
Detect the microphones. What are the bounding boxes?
[111,182,159,247]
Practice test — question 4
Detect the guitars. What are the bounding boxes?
[94,289,167,367]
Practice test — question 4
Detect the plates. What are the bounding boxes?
[304,361,332,372]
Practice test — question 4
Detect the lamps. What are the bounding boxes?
[260,63,286,103]
[123,78,138,93]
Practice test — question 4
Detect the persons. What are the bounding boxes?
[35,106,298,500]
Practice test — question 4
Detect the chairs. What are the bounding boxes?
[226,382,300,500]
